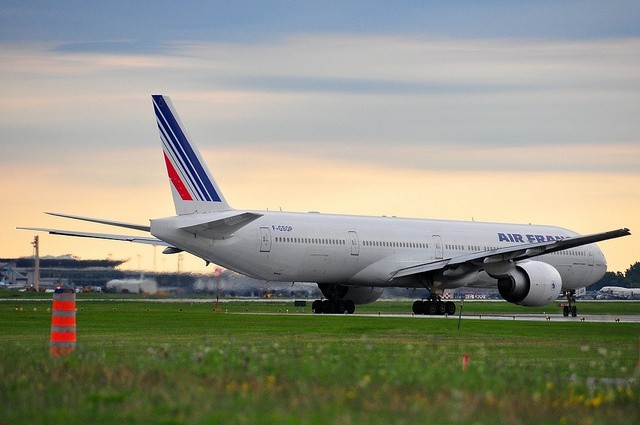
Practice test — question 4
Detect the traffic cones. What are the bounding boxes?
[50,288,76,356]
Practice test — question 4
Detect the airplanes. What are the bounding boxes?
[17,94,631,317]
[594,287,640,300]
